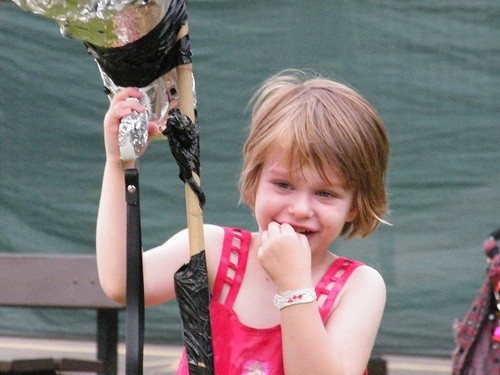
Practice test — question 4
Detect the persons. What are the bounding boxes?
[96,68,392,375]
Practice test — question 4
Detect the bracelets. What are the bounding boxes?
[273,287,317,311]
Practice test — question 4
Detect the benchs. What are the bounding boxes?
[0,253,124,375]
